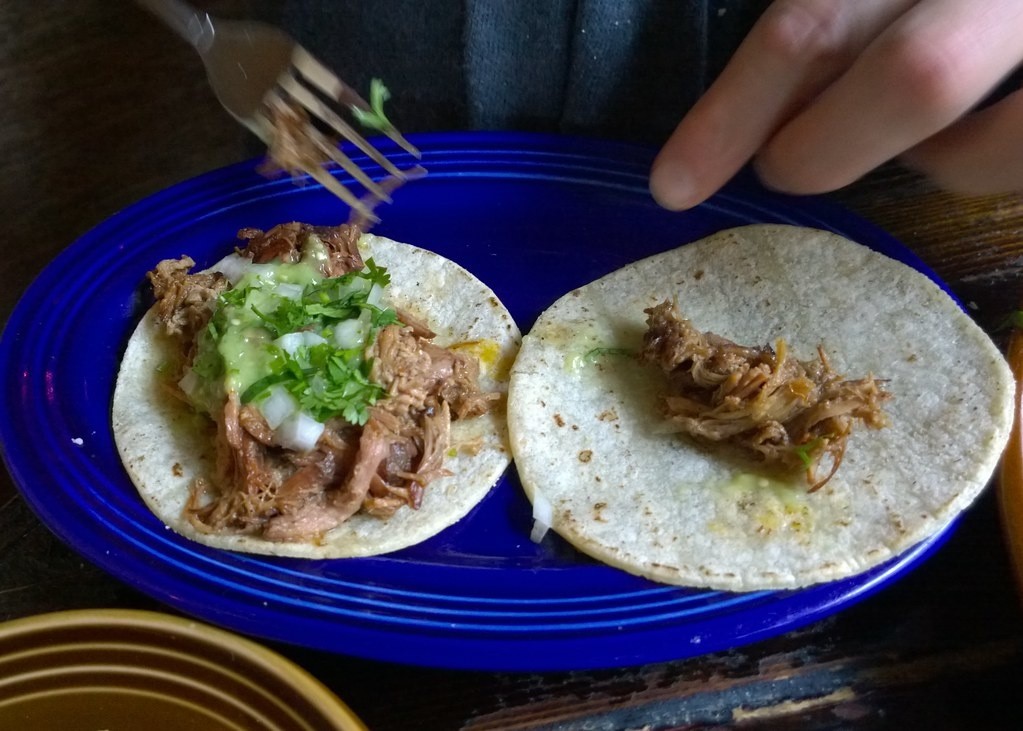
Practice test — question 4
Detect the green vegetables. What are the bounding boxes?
[153,77,406,428]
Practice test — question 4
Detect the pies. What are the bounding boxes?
[507,221,1018,591]
[111,231,524,561]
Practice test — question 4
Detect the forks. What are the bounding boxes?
[136,0,421,224]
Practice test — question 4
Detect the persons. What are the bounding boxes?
[276,0,1022,214]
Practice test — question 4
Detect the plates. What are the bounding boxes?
[0,130,971,670]
[0,609,369,731]
